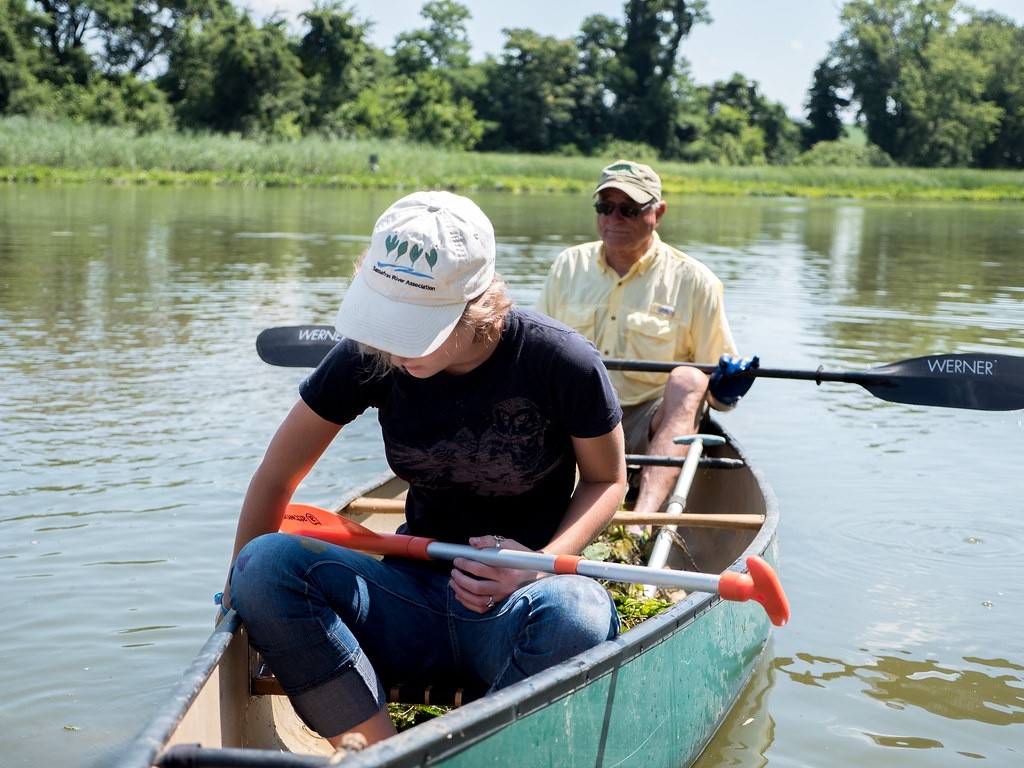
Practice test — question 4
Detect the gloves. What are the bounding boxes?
[707,355,761,414]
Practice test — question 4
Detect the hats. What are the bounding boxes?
[334,190,496,359]
[592,159,662,204]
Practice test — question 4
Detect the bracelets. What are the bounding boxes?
[215,592,229,616]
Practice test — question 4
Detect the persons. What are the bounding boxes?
[215,190,630,766]
[530,160,760,546]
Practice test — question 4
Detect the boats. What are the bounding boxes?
[148,405,782,768]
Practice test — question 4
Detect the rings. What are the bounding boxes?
[494,535,505,547]
[485,596,494,608]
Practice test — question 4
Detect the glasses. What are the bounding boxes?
[594,198,655,217]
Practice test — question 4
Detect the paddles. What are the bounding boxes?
[255,324,1024,412]
[278,504,792,628]
